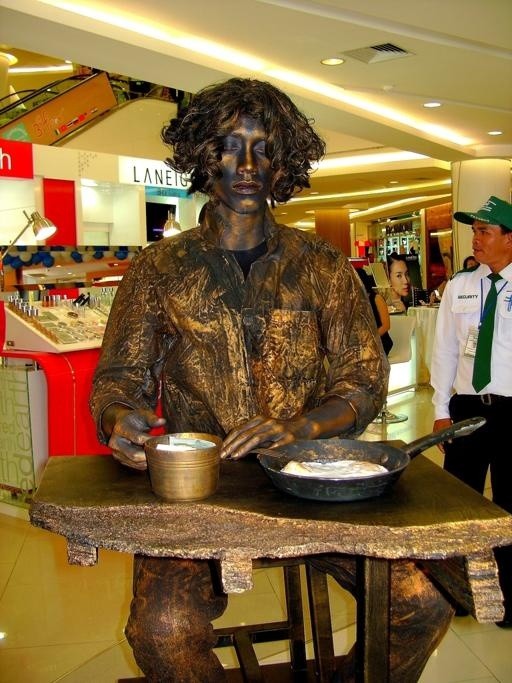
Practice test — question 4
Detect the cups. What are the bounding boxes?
[143,432,224,508]
[9,295,39,318]
[42,294,68,307]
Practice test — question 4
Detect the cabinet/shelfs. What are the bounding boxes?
[375,208,427,291]
[0,137,210,246]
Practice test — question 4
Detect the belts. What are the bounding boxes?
[457,393,506,405]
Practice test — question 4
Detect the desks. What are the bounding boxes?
[28,439,512,683]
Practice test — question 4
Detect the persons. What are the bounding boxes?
[387,256,411,311]
[356,269,393,356]
[429,196,512,628]
[88,76,457,683]
[464,256,479,269]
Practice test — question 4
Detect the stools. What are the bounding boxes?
[209,555,308,683]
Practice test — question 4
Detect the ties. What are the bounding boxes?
[471,271,503,392]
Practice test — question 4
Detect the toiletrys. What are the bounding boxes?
[42,287,115,308]
[8,295,38,323]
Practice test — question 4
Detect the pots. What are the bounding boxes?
[261,413,488,506]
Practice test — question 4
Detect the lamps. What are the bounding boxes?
[0,210,59,259]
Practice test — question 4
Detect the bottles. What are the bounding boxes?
[101,286,114,306]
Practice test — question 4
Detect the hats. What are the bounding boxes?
[451,193,511,230]
